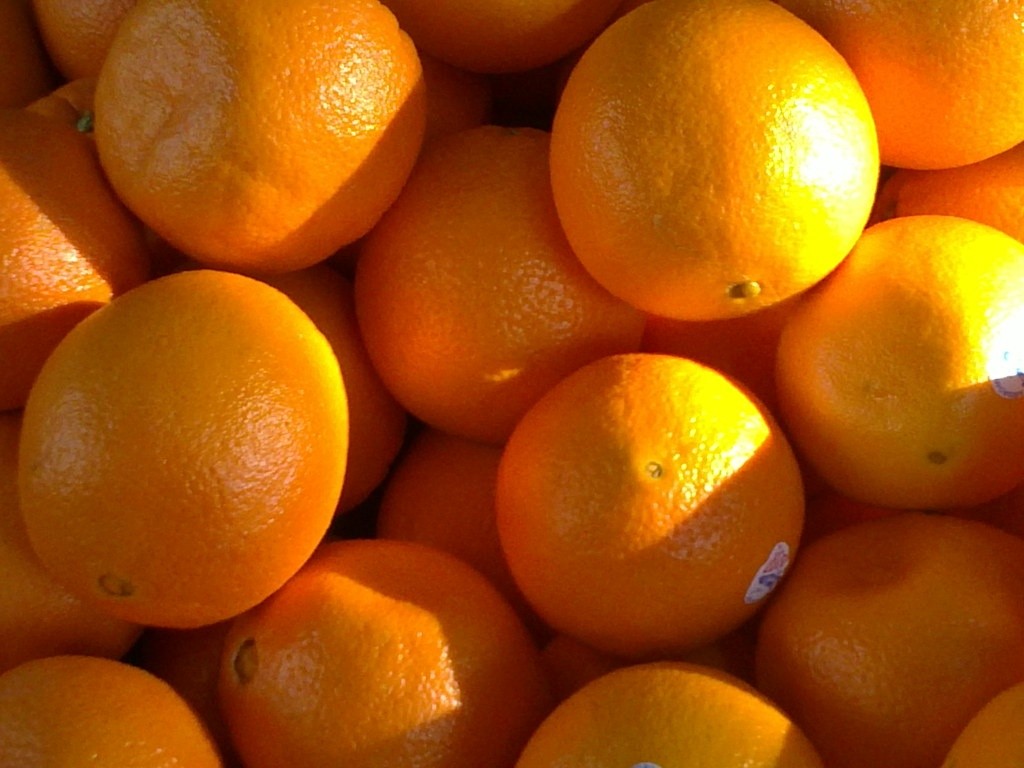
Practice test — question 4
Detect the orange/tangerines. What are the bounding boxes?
[0,2,1023,768]
